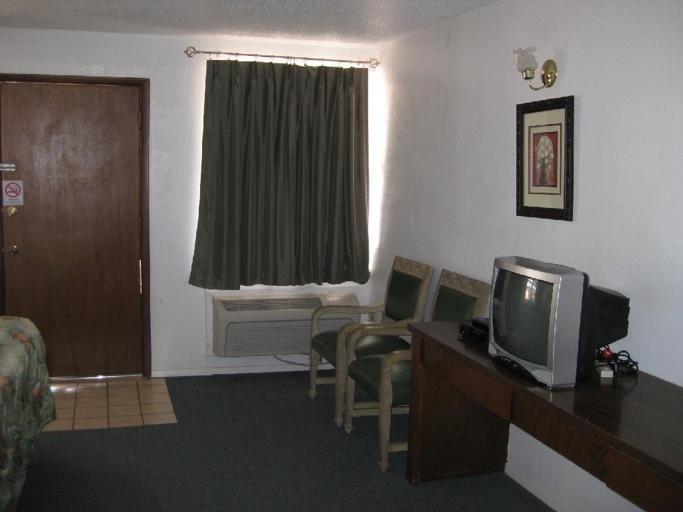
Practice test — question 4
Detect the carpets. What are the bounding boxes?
[40,376,178,433]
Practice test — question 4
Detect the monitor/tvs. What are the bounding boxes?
[488,255,630,388]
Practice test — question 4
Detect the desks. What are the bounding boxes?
[405,316,682,511]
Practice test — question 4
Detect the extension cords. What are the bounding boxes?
[594,356,613,378]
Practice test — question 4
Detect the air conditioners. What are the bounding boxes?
[212,294,361,359]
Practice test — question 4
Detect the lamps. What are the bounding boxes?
[513,44,557,90]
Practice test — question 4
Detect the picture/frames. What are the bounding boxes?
[515,94,574,222]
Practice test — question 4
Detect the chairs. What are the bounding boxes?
[310,254,435,428]
[345,268,492,473]
